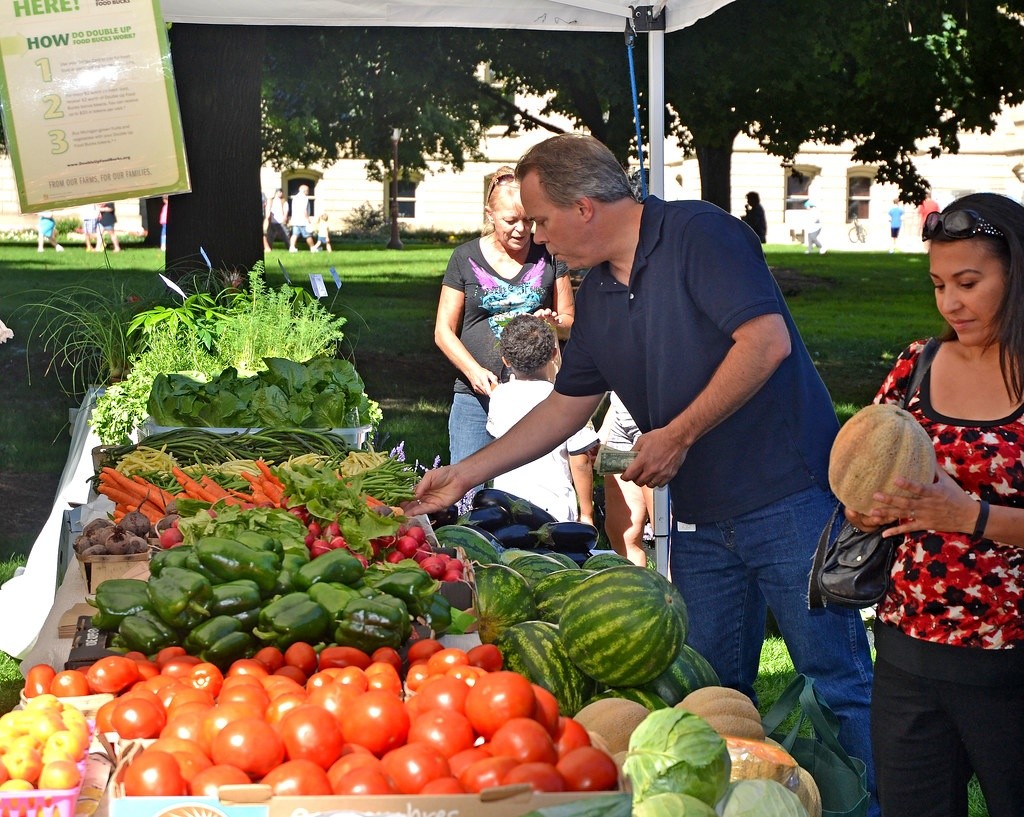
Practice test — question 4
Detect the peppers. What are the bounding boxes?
[85,530,451,672]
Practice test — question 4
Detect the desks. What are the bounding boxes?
[54,383,104,486]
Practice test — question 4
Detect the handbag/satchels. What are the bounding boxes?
[762,675,869,816]
[809,337,943,608]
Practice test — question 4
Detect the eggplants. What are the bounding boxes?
[427,489,599,568]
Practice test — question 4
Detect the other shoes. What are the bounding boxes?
[288,247,298,252]
[311,246,319,253]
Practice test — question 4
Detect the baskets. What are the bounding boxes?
[0,712,95,817]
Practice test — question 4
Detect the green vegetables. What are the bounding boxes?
[146,355,383,428]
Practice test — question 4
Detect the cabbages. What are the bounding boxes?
[631,792,719,817]
[722,778,811,817]
[623,707,731,808]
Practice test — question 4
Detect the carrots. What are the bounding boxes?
[97,460,288,527]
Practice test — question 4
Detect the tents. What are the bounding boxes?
[155,0,736,575]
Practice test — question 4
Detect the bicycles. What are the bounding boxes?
[847,212,868,244]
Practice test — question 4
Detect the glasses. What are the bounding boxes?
[924,208,1003,240]
[488,174,514,203]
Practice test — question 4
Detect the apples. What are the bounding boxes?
[0,693,91,817]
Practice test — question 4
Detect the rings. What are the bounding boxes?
[417,499,422,505]
[909,510,914,519]
[650,480,660,487]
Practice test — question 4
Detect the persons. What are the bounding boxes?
[434,168,577,518]
[596,389,675,583]
[485,315,600,530]
[160,196,168,250]
[803,199,828,255]
[736,191,766,244]
[398,132,883,817]
[848,190,1024,817]
[917,193,941,250]
[34,211,64,253]
[260,183,333,254]
[79,200,120,254]
[886,199,905,253]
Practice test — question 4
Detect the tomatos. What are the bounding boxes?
[0,642,617,795]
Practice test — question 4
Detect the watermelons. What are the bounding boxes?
[435,527,721,710]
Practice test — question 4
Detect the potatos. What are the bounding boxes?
[74,502,182,555]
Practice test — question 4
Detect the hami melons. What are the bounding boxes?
[573,686,822,817]
[828,403,934,516]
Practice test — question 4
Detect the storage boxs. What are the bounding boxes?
[54,411,626,817]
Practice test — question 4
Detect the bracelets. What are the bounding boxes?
[970,499,990,541]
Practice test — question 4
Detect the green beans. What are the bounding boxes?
[86,426,421,506]
